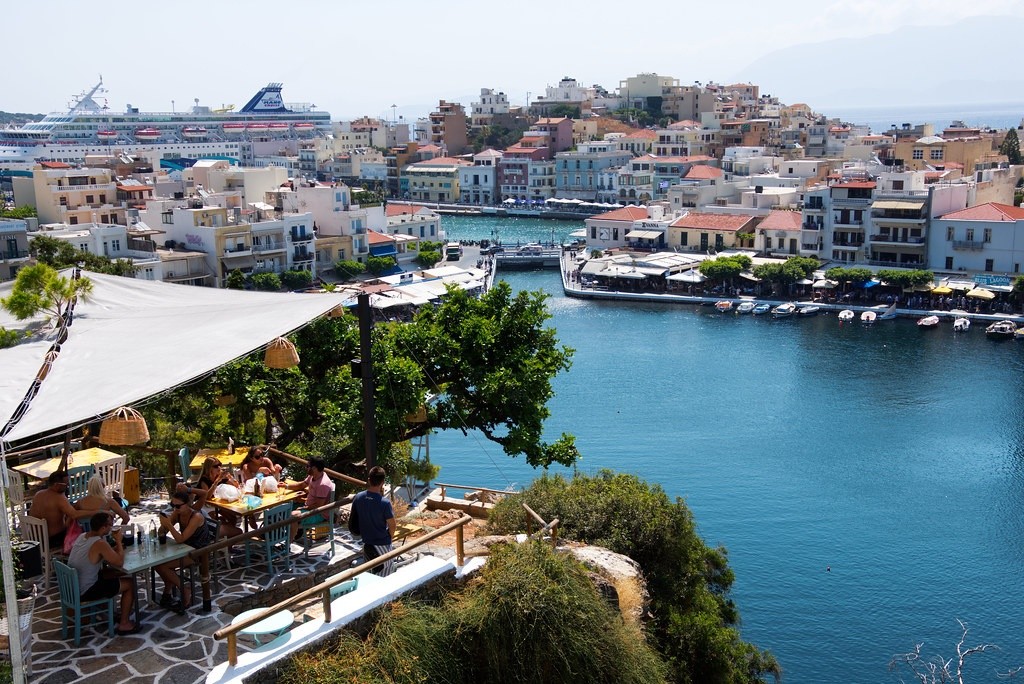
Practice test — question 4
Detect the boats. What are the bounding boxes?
[838,309,854,322]
[771,302,795,319]
[794,306,819,316]
[877,303,896,320]
[1014,327,1024,339]
[917,316,939,329]
[715,300,733,313]
[986,320,1017,338]
[736,302,755,314]
[954,316,971,332]
[861,311,876,324]
[752,304,770,315]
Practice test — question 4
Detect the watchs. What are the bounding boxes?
[286,484,288,489]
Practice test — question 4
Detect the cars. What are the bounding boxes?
[480,245,505,255]
[521,243,543,251]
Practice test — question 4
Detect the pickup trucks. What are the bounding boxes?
[563,240,586,251]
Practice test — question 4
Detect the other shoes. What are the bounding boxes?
[218,547,242,569]
[159,593,174,605]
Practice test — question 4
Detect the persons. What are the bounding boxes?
[639,277,689,293]
[158,491,209,609]
[29,471,130,547]
[569,251,576,261]
[264,456,333,555]
[175,482,243,562]
[886,289,1014,314]
[192,457,243,555]
[65,512,143,636]
[347,466,396,574]
[567,269,587,290]
[818,289,869,307]
[240,446,282,528]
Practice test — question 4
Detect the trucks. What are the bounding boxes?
[446,242,460,260]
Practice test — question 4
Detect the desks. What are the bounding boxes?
[189,448,251,483]
[205,479,309,568]
[232,606,295,647]
[107,531,196,627]
[11,447,123,501]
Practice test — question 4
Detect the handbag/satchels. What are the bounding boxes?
[64,518,82,554]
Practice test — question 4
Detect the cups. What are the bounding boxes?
[111,526,121,540]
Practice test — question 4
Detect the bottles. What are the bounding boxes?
[254,479,260,497]
[149,519,157,542]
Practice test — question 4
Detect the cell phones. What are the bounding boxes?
[159,512,167,518]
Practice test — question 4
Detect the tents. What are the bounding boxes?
[665,269,708,283]
[795,275,881,295]
[902,284,998,300]
[594,268,649,279]
[0,263,378,684]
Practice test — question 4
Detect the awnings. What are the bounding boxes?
[871,199,925,210]
[581,262,608,274]
[370,244,399,257]
[625,229,664,239]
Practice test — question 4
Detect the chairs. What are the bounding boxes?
[5,461,335,648]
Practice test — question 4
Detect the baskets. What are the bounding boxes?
[0,584,37,636]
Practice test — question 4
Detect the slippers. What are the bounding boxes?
[95,614,144,636]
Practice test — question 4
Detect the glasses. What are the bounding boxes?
[170,501,185,509]
[211,463,222,468]
[253,453,263,460]
[307,464,311,468]
